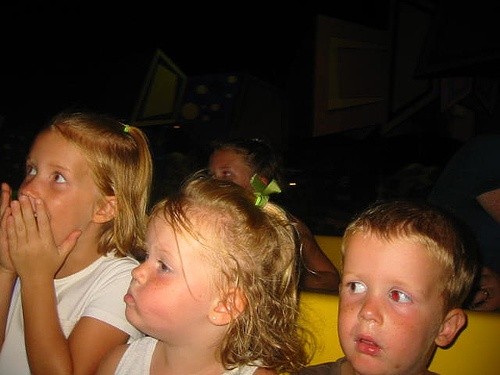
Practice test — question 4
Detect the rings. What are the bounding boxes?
[483,288,490,298]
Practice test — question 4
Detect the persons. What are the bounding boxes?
[208,138,341,291]
[0,112,153,375]
[113,168,316,375]
[427,132,500,312]
[297,197,479,375]
